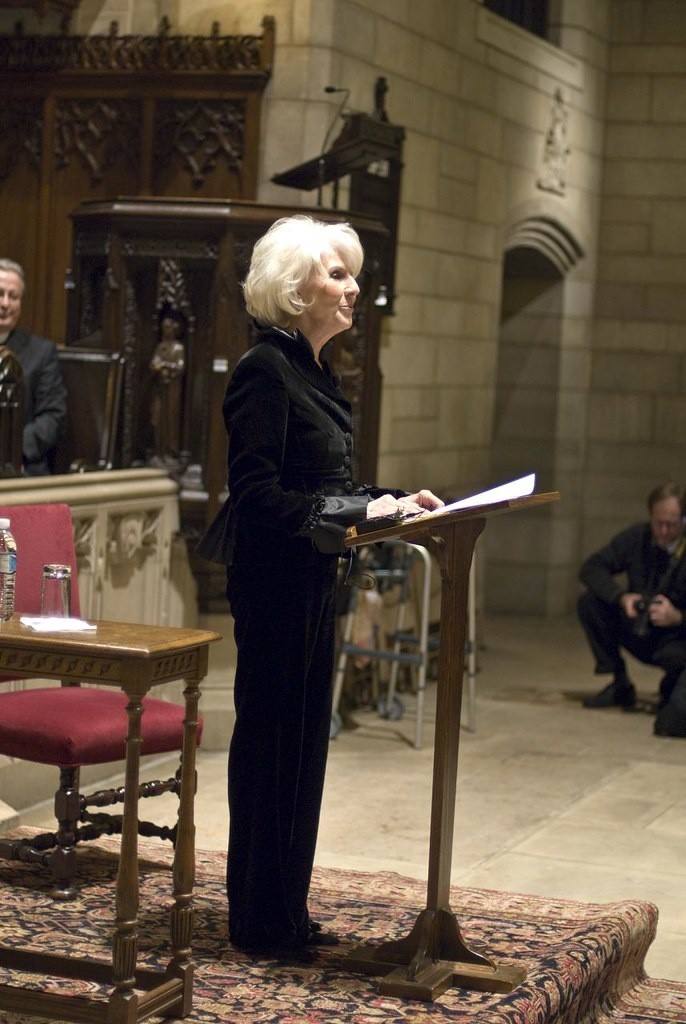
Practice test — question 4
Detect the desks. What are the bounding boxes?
[0,609,220,1024]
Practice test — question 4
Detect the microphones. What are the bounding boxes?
[331,374,341,389]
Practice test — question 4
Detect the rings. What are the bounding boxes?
[397,506,404,512]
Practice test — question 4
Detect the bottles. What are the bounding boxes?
[0,517,17,621]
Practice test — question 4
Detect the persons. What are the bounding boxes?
[150,318,184,458]
[0,258,68,475]
[575,480,686,712]
[194,212,444,960]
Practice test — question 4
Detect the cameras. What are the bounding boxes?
[631,598,662,638]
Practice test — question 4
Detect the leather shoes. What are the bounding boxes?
[230,926,339,960]
[584,683,637,708]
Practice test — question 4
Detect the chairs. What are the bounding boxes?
[0,505,203,903]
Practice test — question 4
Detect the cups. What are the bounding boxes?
[40,565,72,618]
